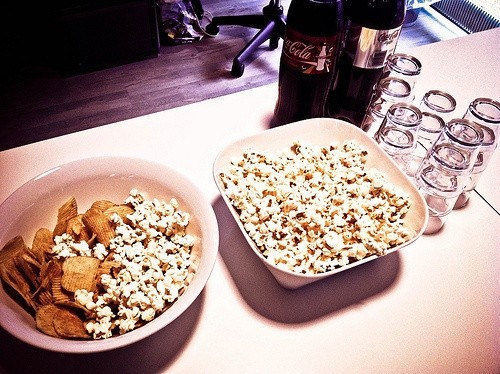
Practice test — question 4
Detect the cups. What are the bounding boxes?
[360,52,500,236]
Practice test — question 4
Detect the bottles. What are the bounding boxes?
[273,0,407,132]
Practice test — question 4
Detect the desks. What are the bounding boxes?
[0,26,500,374]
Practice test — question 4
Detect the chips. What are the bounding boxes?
[0,194,137,338]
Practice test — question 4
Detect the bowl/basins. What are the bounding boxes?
[212,117,429,291]
[0,156,219,354]
